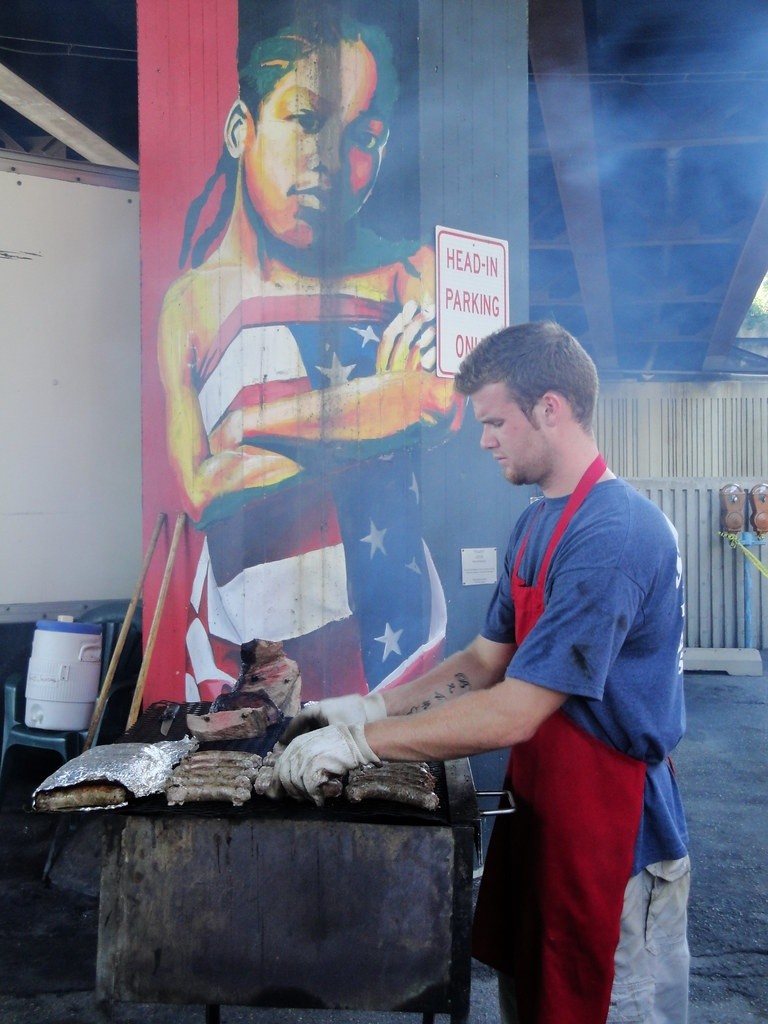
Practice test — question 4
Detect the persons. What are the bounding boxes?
[271,315,692,1024]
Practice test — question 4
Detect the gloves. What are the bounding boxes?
[279,693,387,745]
[273,722,383,806]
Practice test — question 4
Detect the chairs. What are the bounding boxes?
[2,618,119,787]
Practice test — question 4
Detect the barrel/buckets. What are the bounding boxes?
[24,618,103,731]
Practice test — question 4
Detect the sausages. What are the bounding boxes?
[34,746,441,810]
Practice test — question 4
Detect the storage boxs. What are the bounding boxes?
[24,620,108,731]
